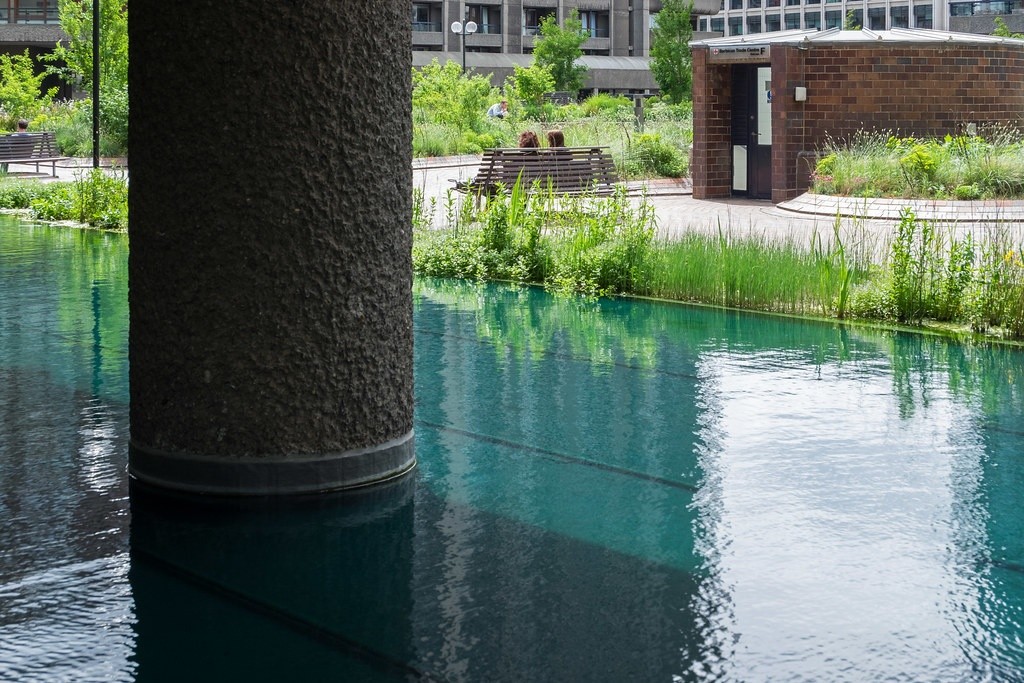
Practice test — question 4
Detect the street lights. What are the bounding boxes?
[451,19,478,75]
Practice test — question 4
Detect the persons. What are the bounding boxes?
[6,119,34,158]
[487,101,508,119]
[545,131,573,161]
[512,131,540,162]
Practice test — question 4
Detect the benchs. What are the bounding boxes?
[453,148,600,193]
[0,133,69,176]
[495,148,630,208]
[542,92,571,107]
